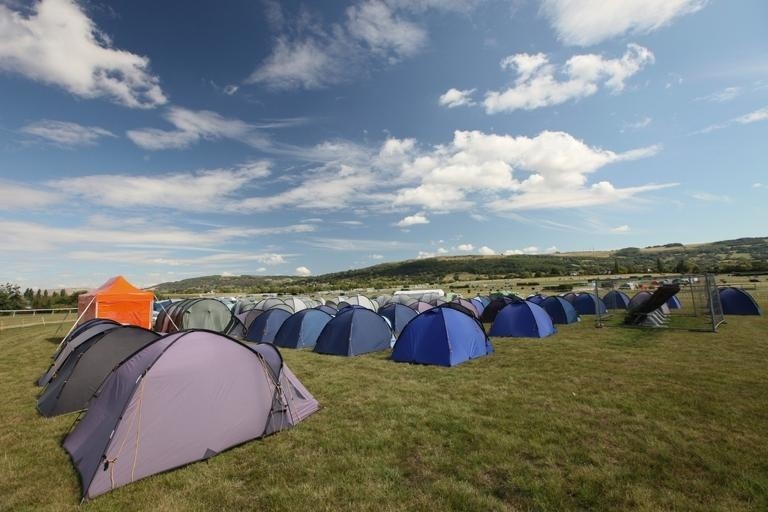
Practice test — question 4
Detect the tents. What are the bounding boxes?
[29,324,161,421]
[152,289,578,369]
[56,330,328,510]
[73,273,154,330]
[708,287,760,315]
[32,316,122,387]
[571,290,681,315]
